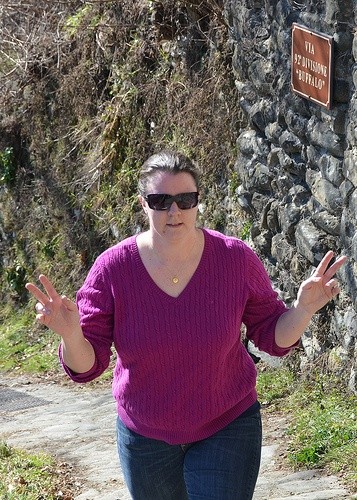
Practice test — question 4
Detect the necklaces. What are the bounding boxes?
[148,229,195,284]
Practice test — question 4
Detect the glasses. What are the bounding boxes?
[142,189,200,211]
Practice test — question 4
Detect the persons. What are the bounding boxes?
[25,150,346,500]
